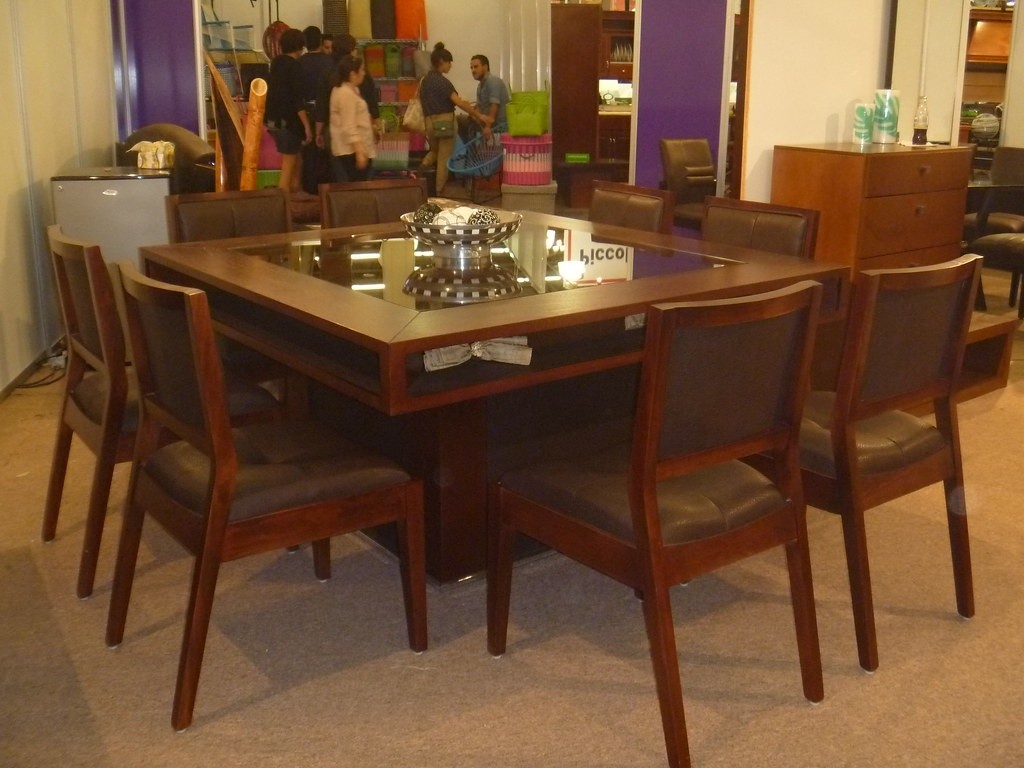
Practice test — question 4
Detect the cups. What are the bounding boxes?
[851,103,875,144]
[873,88,900,143]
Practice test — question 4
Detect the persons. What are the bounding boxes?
[420,41,495,198]
[264,26,380,195]
[470,55,509,142]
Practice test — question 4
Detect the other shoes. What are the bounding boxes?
[408,170,419,178]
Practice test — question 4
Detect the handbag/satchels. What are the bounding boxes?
[371,140,411,170]
[403,75,426,133]
[412,41,432,79]
[506,96,546,138]
[353,42,415,78]
[431,119,454,138]
[508,78,550,132]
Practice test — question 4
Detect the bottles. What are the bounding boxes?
[609,43,633,63]
[911,95,929,145]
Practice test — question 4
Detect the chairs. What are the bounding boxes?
[42,138,1024,768]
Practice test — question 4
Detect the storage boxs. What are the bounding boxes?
[501,78,557,212]
[373,132,409,170]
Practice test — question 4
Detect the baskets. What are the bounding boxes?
[447,123,507,178]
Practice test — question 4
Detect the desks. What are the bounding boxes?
[139,208,852,589]
[965,170,1024,248]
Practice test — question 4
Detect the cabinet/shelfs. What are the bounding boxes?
[551,3,637,193]
[772,142,976,311]
